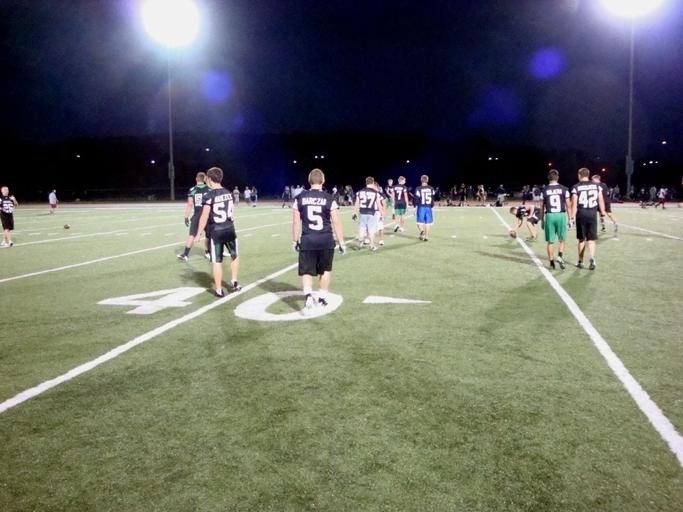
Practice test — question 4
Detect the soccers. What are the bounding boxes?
[64,224,70,229]
[510,230,516,237]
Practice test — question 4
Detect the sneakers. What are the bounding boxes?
[230,280,241,291]
[576,258,595,270]
[419,230,428,241]
[550,256,566,269]
[307,294,327,308]
[359,238,384,251]
[531,233,537,241]
[394,225,402,232]
[614,224,617,232]
[205,252,210,260]
[601,228,605,233]
[178,255,188,261]
[222,252,231,256]
[214,289,223,295]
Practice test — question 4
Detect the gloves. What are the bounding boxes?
[185,217,189,227]
[541,220,545,229]
[291,241,300,253]
[567,217,575,231]
[352,213,359,222]
[600,215,606,224]
[339,244,346,256]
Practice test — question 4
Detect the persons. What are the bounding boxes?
[281,181,675,211]
[539,169,572,272]
[591,173,618,234]
[509,205,541,242]
[570,168,606,270]
[233,186,241,208]
[364,183,386,246]
[244,186,251,207]
[384,178,395,221]
[194,167,242,298]
[412,174,435,242]
[0,185,19,248]
[292,168,346,316]
[48,189,59,214]
[174,171,216,262]
[392,175,409,233]
[251,186,258,208]
[351,176,383,253]
